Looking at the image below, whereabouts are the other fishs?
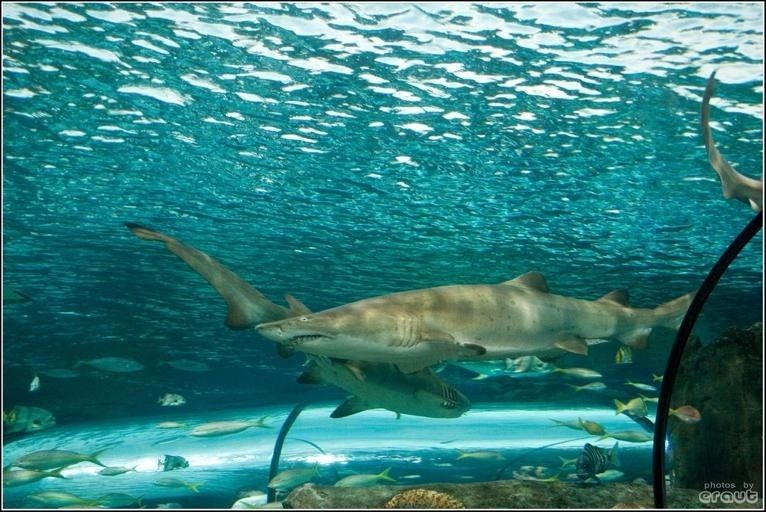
[3,393,208,510]
